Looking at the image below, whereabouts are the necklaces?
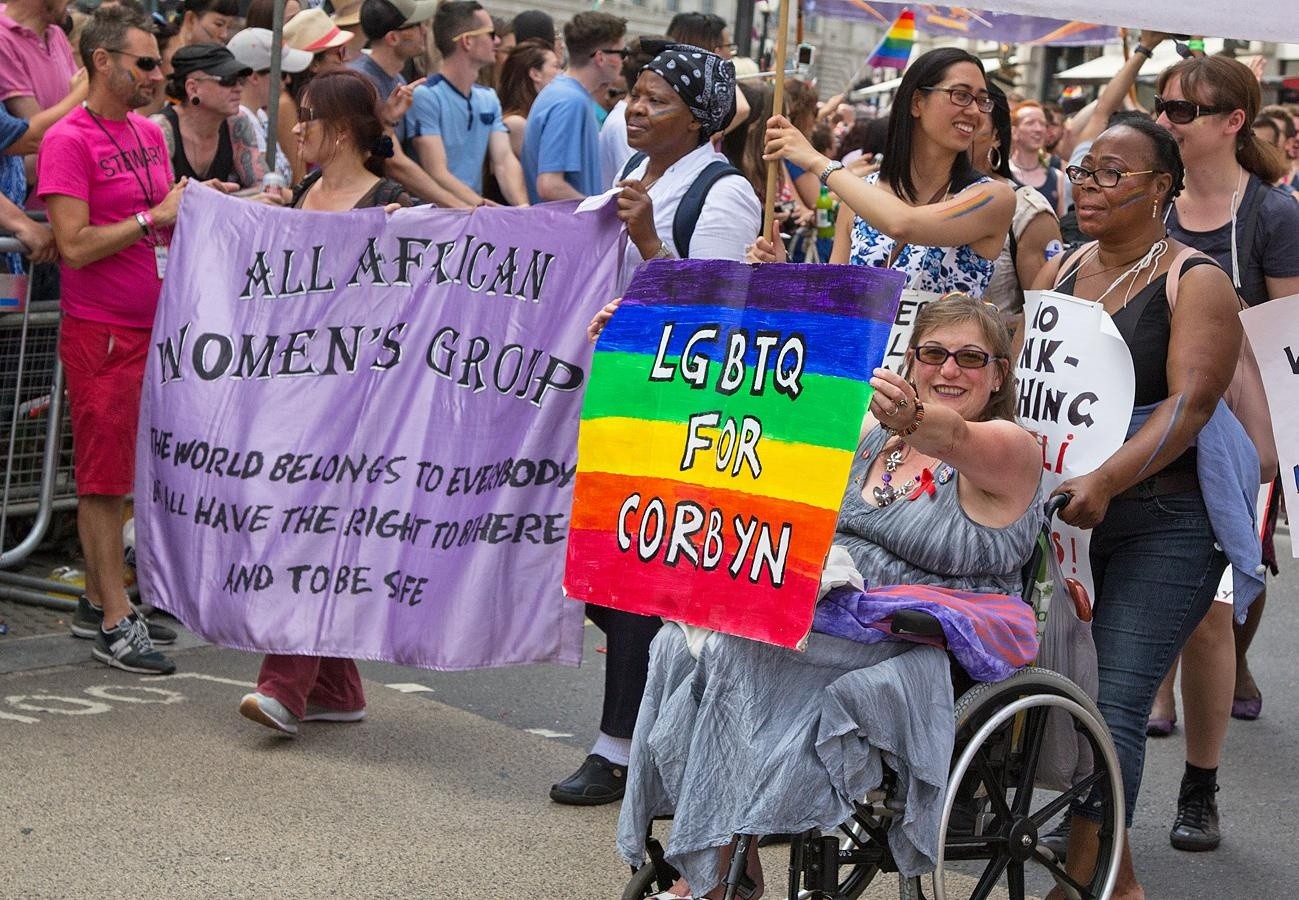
[191,137,210,177]
[1010,157,1040,171]
[872,440,938,506]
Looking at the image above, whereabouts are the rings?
[885,404,899,416]
[894,396,908,408]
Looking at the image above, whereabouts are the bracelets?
[879,396,925,438]
[1188,40,1204,52]
[379,112,400,128]
[1134,45,1153,59]
[142,210,155,229]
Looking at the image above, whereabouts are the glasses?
[920,84,995,113]
[1065,164,1160,188]
[452,27,496,42]
[296,106,325,122]
[911,345,997,368]
[87,47,164,72]
[197,76,244,87]
[589,46,627,60]
[719,42,739,56]
[1154,95,1231,126]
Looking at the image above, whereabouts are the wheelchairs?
[616,487,1127,900]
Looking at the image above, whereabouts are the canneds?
[262,172,286,198]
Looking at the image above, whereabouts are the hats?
[330,0,364,26]
[360,0,437,40]
[282,8,354,53]
[227,28,314,73]
[167,43,253,77]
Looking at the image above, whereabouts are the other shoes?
[1231,689,1262,718]
[1146,713,1177,735]
[551,754,628,806]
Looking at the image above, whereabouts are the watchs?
[135,213,149,236]
[652,241,672,260]
[819,159,845,187]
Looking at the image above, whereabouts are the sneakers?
[1038,807,1072,865]
[297,702,366,722]
[1170,773,1220,851]
[92,613,176,674]
[72,593,177,644]
[239,692,298,736]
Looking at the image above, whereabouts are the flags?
[866,10,916,70]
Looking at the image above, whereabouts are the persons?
[1027,116,1245,900]
[238,65,417,736]
[617,292,1047,899]
[36,5,230,675]
[547,44,764,806]
[0,0,1299,835]
